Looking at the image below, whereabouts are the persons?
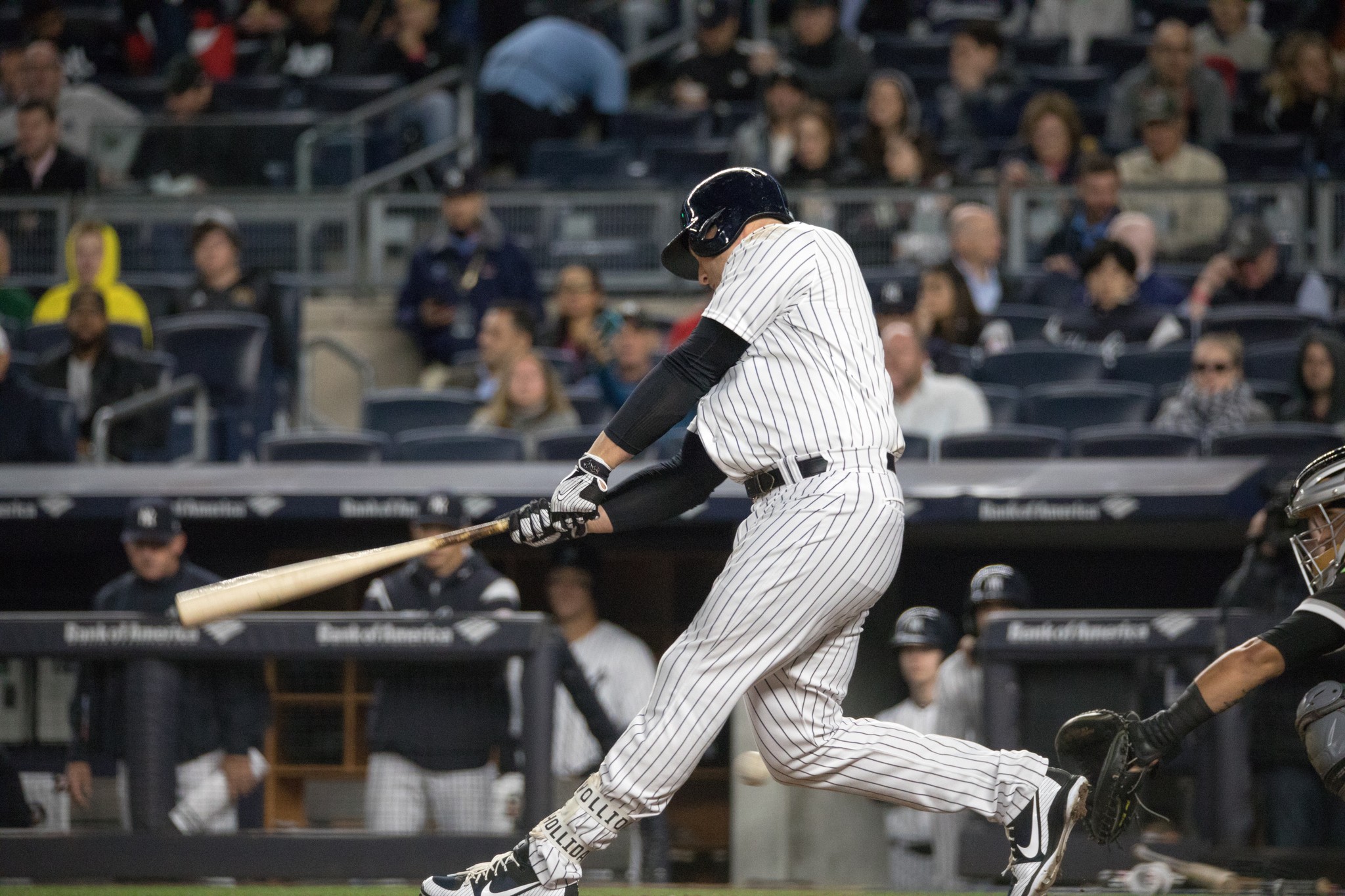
[421,164,1092,896]
[0,0,1345,896]
[62,496,278,833]
[1049,444,1345,896]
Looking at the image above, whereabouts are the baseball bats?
[175,475,610,629]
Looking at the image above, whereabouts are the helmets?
[1284,449,1345,593]
[662,166,794,281]
[969,566,1023,607]
[893,604,952,652]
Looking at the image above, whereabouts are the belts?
[745,452,896,497]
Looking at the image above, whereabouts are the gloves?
[495,499,588,548]
[553,452,615,532]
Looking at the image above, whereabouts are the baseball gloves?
[1055,710,1152,846]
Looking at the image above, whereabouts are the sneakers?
[420,840,579,896]
[1000,765,1093,896]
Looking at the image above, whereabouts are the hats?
[119,496,179,544]
[443,168,480,196]
[410,492,470,530]
